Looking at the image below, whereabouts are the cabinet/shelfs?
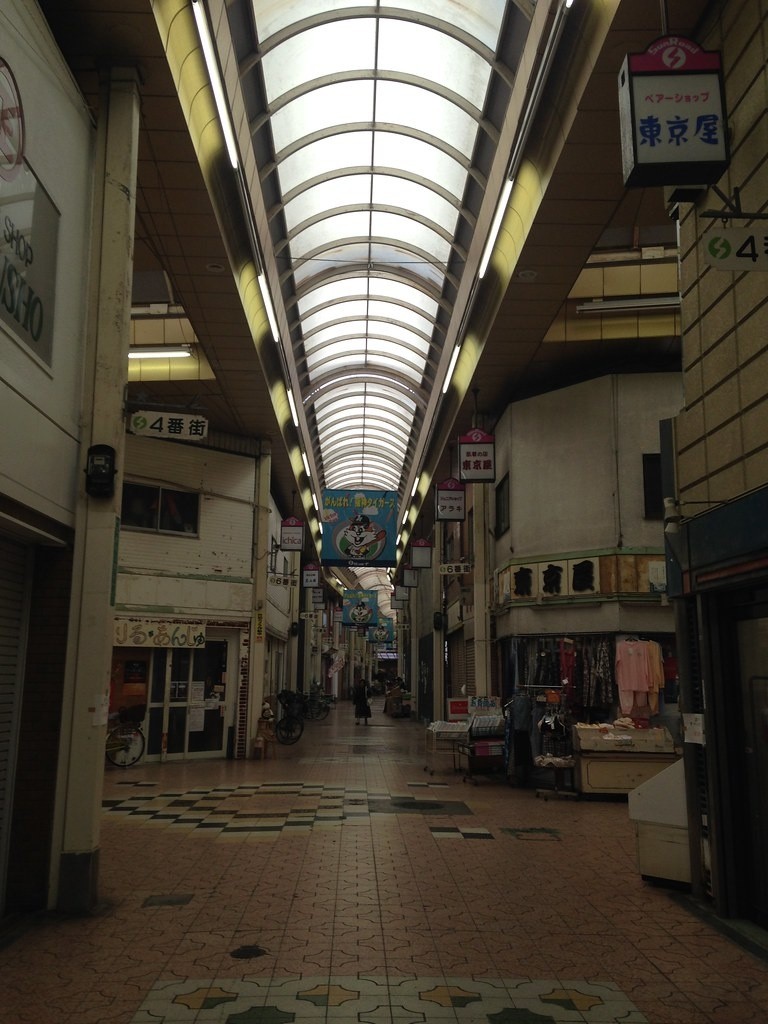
[424,728,504,785]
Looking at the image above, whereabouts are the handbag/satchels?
[367,698,373,706]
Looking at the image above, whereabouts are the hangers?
[582,634,612,643]
[625,633,650,642]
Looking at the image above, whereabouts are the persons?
[386,680,395,690]
[353,679,372,725]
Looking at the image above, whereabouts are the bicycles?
[275,690,330,747]
[106,713,145,768]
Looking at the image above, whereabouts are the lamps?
[84,444,116,502]
[661,494,726,523]
[433,612,443,630]
[292,621,298,636]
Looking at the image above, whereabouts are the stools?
[257,718,276,766]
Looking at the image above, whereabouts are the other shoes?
[356,722,359,725]
[365,722,367,725]
